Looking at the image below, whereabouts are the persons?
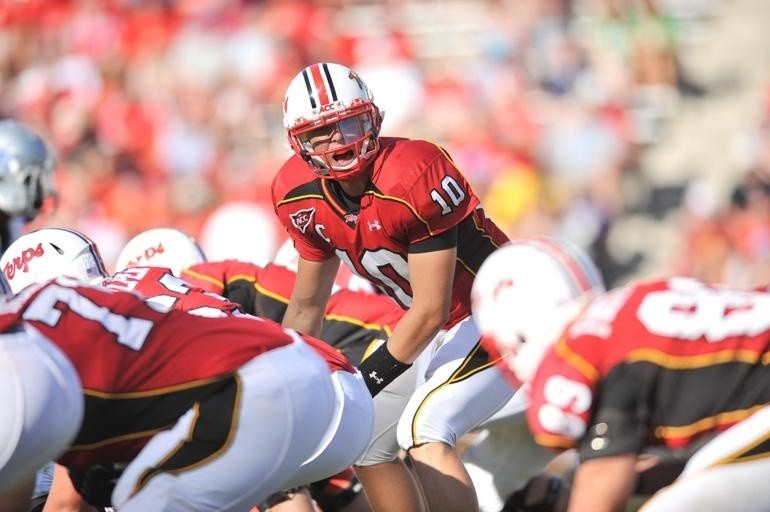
[1,226,377,511]
[267,59,522,512]
[469,234,770,512]
[114,227,430,512]
[0,266,86,512]
[0,2,768,303]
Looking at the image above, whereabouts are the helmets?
[0,119,52,221]
[469,234,608,390]
[0,227,110,302]
[115,227,208,279]
[283,62,381,178]
